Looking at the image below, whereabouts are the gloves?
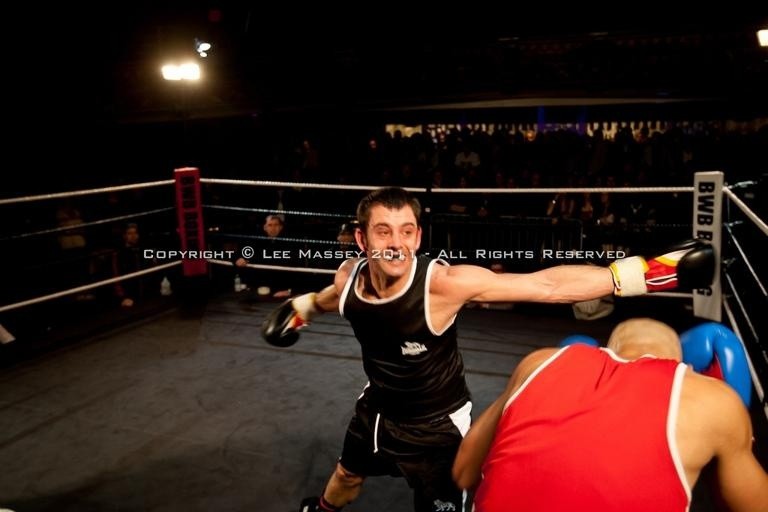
[610,240,716,297]
[681,323,751,405]
[262,292,316,347]
[561,334,598,346]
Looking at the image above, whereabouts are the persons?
[451,318,768,512]
[261,188,715,512]
[57,126,662,344]
